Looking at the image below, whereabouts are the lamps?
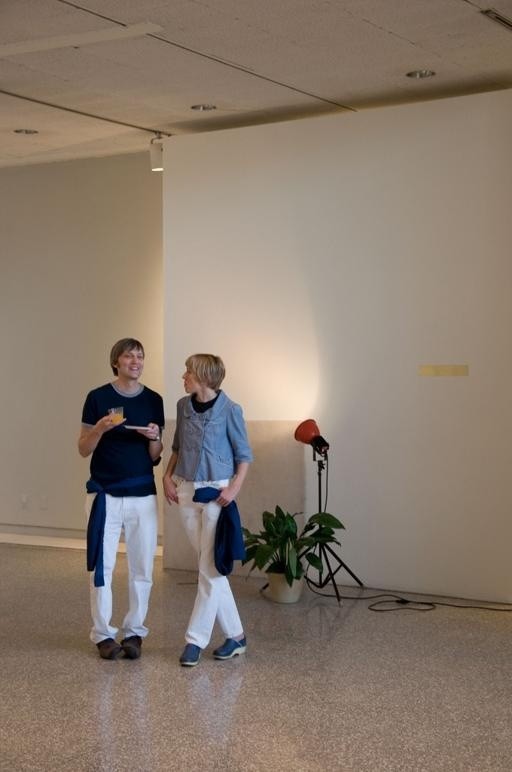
[149,132,163,171]
[259,419,363,602]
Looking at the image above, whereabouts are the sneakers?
[214,635,248,659]
[97,637,122,659]
[179,643,203,666]
[120,635,142,658]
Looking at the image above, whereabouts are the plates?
[125,425,152,431]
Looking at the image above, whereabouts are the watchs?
[150,434,159,441]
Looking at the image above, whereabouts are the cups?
[108,406,124,424]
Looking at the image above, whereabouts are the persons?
[162,354,255,666]
[79,338,166,660]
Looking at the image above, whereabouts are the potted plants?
[240,505,346,601]
